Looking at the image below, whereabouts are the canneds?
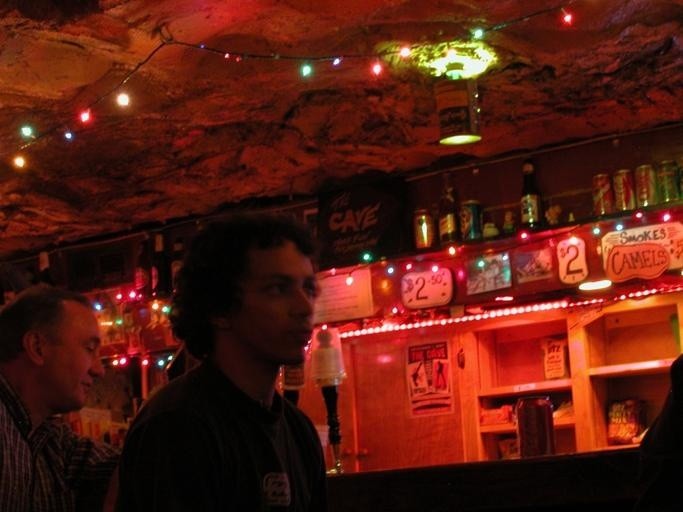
[458,199,482,242]
[515,396,556,458]
[415,209,436,249]
[589,159,682,217]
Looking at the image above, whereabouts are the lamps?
[434,77,483,145]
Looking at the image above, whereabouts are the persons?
[114,209,329,512]
[465,251,511,295]
[0,282,122,510]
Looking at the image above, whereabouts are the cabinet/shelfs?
[334,291,682,476]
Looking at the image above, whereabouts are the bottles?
[31,247,55,289]
[436,169,459,244]
[518,156,542,234]
[132,231,187,303]
